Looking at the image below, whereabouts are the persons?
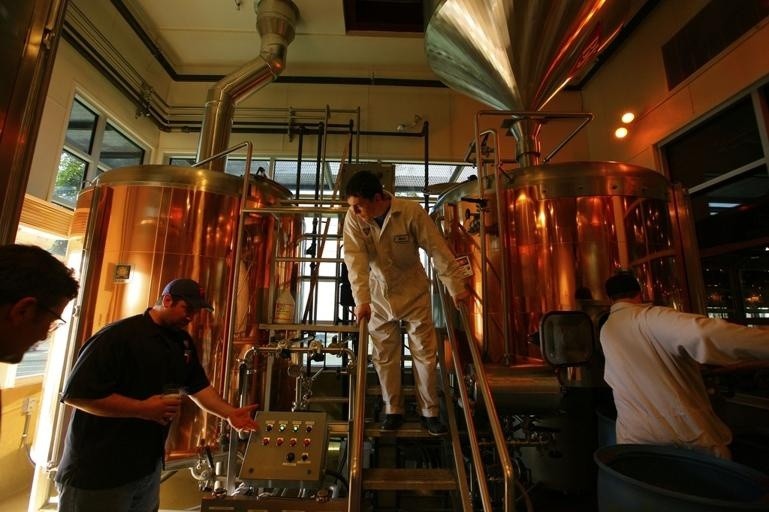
[598,270,769,461]
[574,286,608,445]
[52,278,261,511]
[0,243,80,366]
[342,169,470,437]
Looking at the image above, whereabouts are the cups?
[162,386,182,422]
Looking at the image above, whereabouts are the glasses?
[40,305,67,333]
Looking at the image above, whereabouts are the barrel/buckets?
[592,443,769,512]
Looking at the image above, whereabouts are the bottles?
[273,283,296,325]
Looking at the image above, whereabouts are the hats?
[161,278,214,313]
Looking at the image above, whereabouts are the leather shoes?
[381,414,405,429]
[420,414,450,436]
